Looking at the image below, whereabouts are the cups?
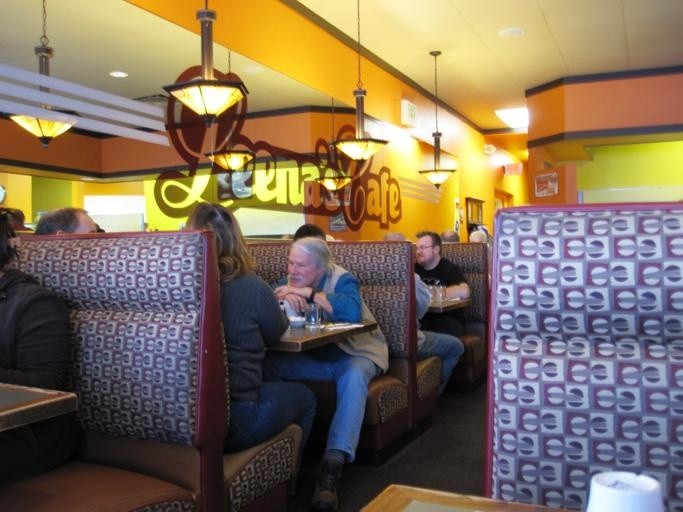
[304,302,322,332]
[280,295,306,331]
[425,283,447,305]
[586,471,664,511]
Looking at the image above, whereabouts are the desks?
[358,484,575,511]
[275,319,379,353]
[1,383,79,433]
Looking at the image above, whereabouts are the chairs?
[485,203,682,511]
[243,240,488,432]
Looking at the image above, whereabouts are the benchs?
[16,230,304,509]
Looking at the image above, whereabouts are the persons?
[385,231,471,393]
[469,226,493,243]
[183,203,318,495]
[0,205,75,483]
[270,237,388,509]
[293,224,326,241]
[35,208,95,235]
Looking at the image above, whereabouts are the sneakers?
[311,458,344,510]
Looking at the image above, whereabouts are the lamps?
[419,51,455,190]
[331,1,389,166]
[162,0,250,128]
[205,50,256,175]
[7,0,81,149]
